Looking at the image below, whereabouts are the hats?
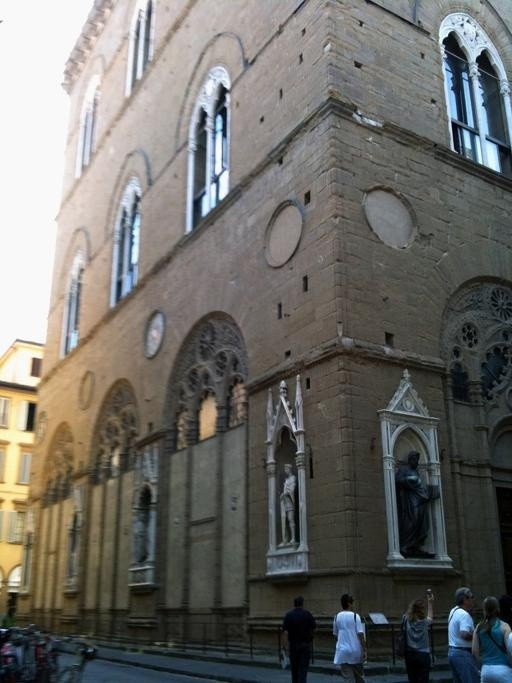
[455,587,474,599]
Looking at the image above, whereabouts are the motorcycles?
[0,624,99,682]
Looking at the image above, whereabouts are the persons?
[445,587,475,683]
[280,595,319,683]
[400,592,435,683]
[394,450,438,556]
[471,596,512,683]
[332,592,369,683]
[277,463,297,547]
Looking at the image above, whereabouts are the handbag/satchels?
[394,634,409,657]
